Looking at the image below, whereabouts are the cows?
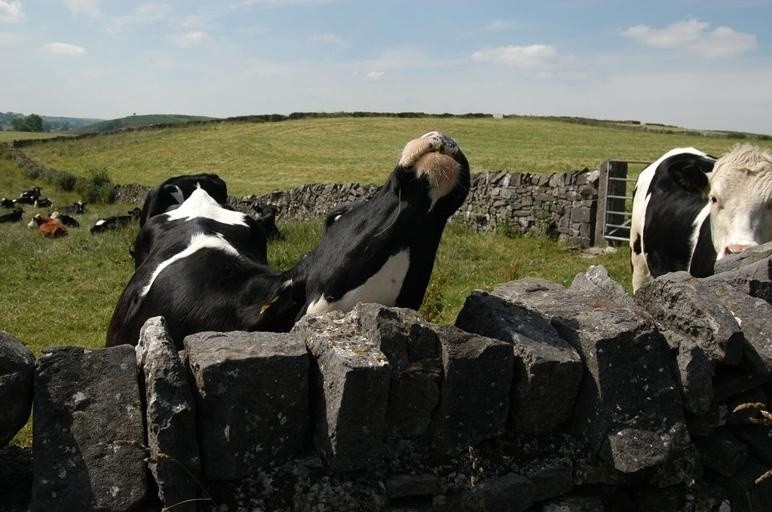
[27,214,69,240]
[104,130,471,348]
[54,200,88,218]
[0,185,56,223]
[629,142,772,295]
[139,172,228,228]
[89,207,142,236]
[50,211,80,231]
